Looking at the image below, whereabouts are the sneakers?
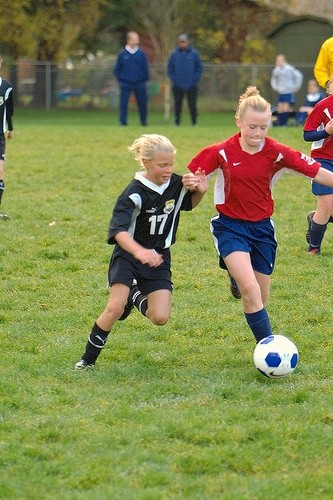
[118,278,138,320]
[228,273,242,299]
[75,360,94,372]
[306,211,316,243]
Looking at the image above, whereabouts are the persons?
[0,57,14,220]
[113,31,149,126]
[270,53,304,127]
[303,93,333,255]
[181,86,333,344]
[296,80,328,126]
[75,134,208,372]
[313,37,333,96]
[168,33,202,127]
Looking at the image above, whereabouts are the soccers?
[254,334,299,378]
